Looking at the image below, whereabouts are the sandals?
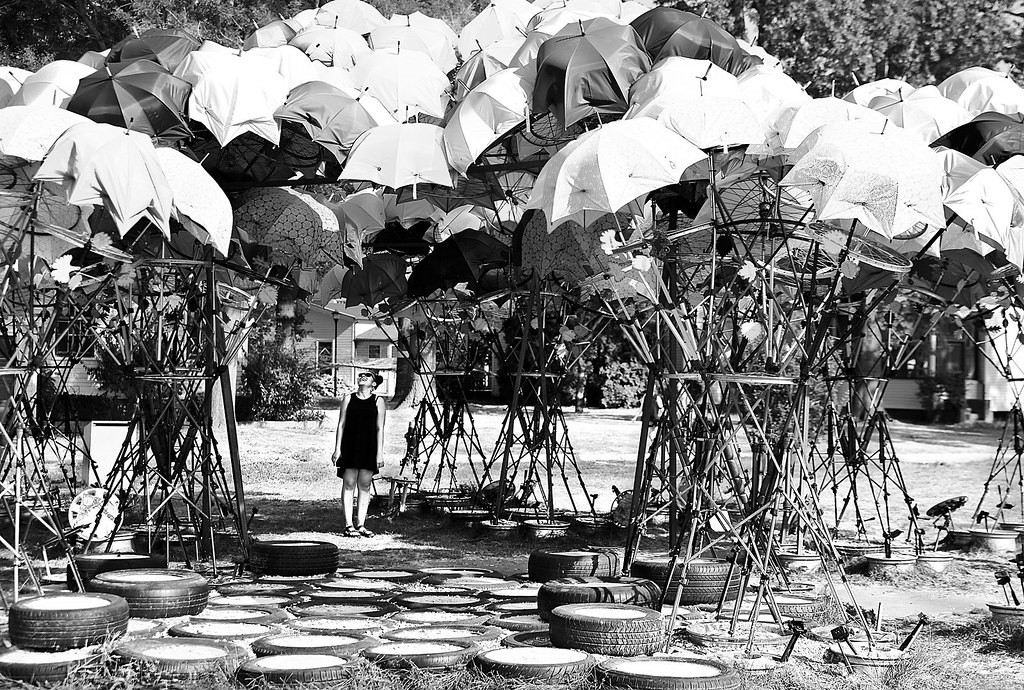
[343,524,361,538]
[355,525,375,538]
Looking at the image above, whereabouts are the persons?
[332,370,383,536]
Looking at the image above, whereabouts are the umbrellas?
[1,0,1023,319]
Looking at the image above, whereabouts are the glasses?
[358,372,376,382]
